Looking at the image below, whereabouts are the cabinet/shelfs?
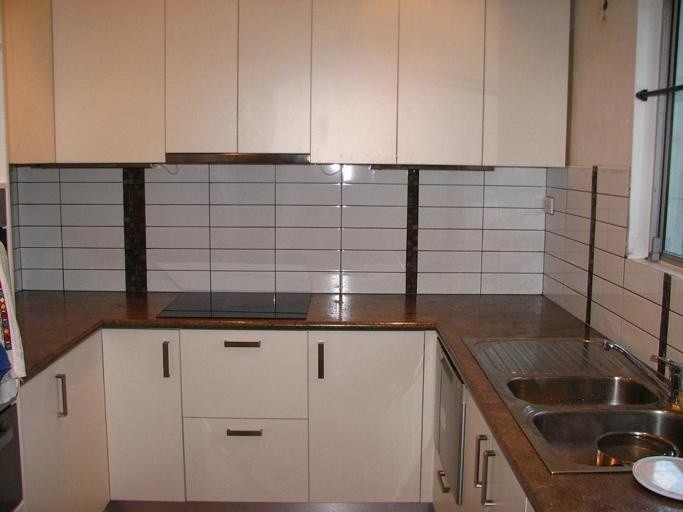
[397,1,570,169]
[180,326,309,503]
[101,327,186,502]
[165,1,312,153]
[15,327,109,512]
[420,329,533,512]
[309,2,399,165]
[308,328,425,503]
[2,2,166,164]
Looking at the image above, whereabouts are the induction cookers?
[154,292,312,320]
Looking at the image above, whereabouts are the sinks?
[532,409,683,466]
[508,374,662,405]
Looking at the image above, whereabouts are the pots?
[593,430,680,467]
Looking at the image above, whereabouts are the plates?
[632,454,683,502]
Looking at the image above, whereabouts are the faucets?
[601,340,683,403]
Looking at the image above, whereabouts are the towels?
[0,239,26,407]
